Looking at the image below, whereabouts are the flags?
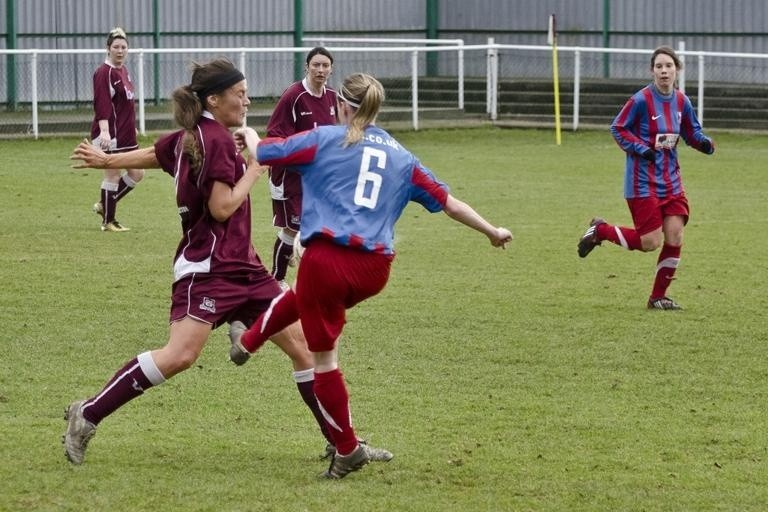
[547,15,555,47]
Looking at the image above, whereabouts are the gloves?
[642,149,655,164]
[698,139,711,154]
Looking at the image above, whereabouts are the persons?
[578,46,713,307]
[63,59,396,467]
[255,45,346,291]
[91,26,144,234]
[228,74,512,479]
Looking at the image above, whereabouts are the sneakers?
[319,445,370,479]
[93,203,105,217]
[288,234,302,267]
[578,217,608,258]
[61,400,97,466]
[229,320,251,366]
[101,221,129,231]
[277,280,290,292]
[647,297,681,310]
[325,435,394,461]
